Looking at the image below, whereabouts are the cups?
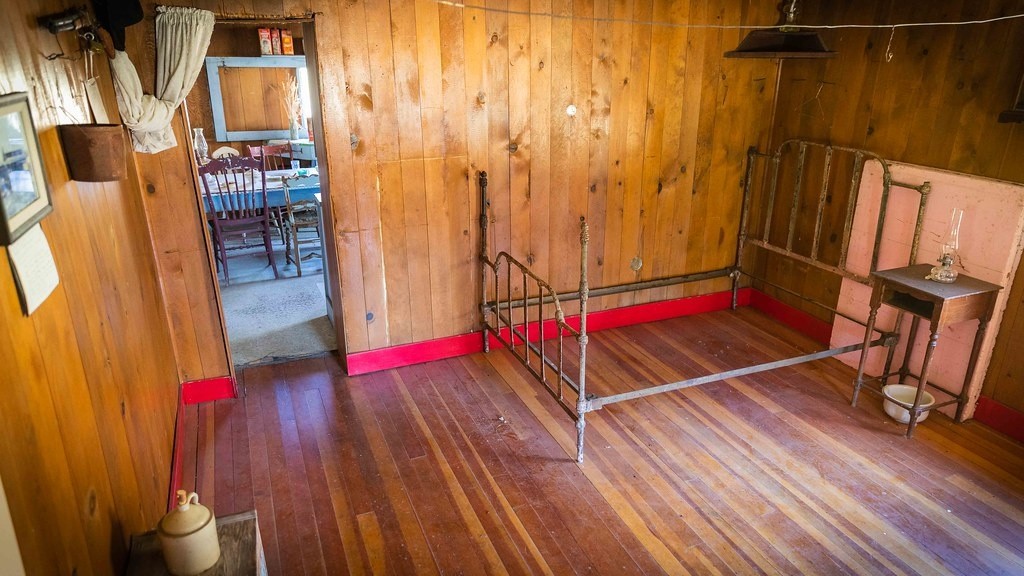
[291,160,300,171]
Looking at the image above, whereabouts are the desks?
[268,137,318,171]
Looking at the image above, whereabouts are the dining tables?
[196,167,322,216]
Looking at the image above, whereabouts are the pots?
[155,488,221,574]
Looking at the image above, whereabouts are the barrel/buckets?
[58,105,130,182]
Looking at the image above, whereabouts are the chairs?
[198,149,282,288]
[201,142,282,247]
[279,174,325,277]
[248,142,321,243]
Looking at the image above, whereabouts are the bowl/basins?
[880,382,936,423]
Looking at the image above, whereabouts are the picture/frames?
[0,89,55,246]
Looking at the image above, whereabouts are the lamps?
[721,1,842,62]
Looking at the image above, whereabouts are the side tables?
[850,262,1007,440]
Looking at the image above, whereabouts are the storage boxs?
[54,80,129,183]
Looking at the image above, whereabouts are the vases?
[192,127,209,161]
[288,117,299,140]
